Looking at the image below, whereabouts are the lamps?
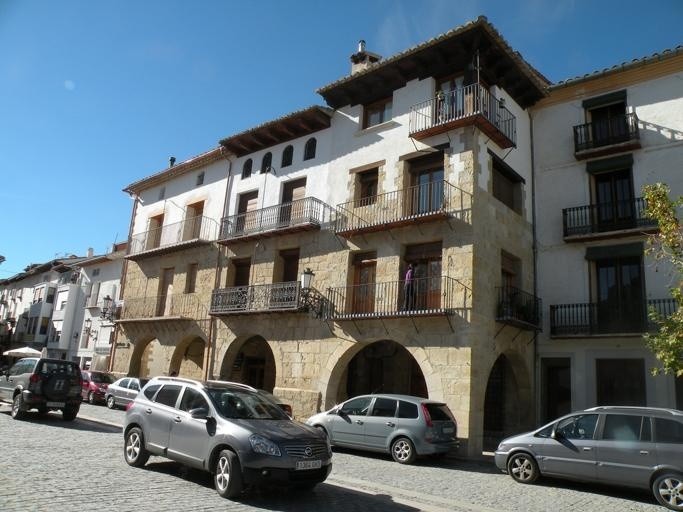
[83,318,97,342]
[99,295,119,329]
[300,267,328,320]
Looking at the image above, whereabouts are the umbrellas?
[2,346,40,359]
[41,347,48,372]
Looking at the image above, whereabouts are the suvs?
[0,357,83,422]
[123,375,332,497]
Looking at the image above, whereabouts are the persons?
[400,262,417,310]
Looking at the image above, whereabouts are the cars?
[492,406,683,511]
[81,370,118,405]
[304,393,460,462]
[105,376,149,409]
